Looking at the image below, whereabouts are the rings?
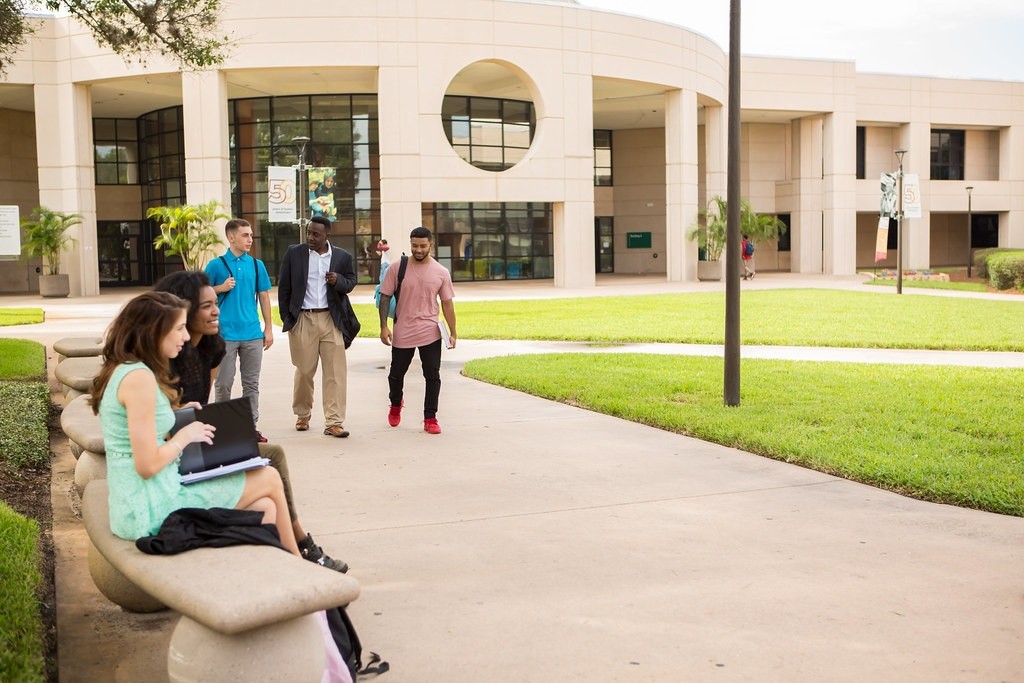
[327,279,328,281]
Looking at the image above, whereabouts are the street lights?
[291,135,311,245]
[894,147,907,293]
[965,186,974,278]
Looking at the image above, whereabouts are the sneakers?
[423,418,441,433]
[254,431,267,443]
[298,533,348,574]
[389,401,405,426]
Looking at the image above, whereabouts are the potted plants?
[739,206,787,278]
[145,200,232,277]
[686,195,758,282]
[11,206,84,298]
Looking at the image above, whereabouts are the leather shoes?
[324,426,348,437]
[296,414,311,430]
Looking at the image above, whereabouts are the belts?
[301,307,329,313]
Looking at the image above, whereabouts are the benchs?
[52,335,359,683]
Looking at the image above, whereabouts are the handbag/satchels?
[373,253,409,317]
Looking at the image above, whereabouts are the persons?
[376,239,390,284]
[84,291,304,560]
[378,227,456,434]
[203,218,274,443]
[308,169,338,222]
[277,216,361,438]
[152,270,349,575]
[740,234,757,282]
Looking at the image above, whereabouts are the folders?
[169,396,266,485]
